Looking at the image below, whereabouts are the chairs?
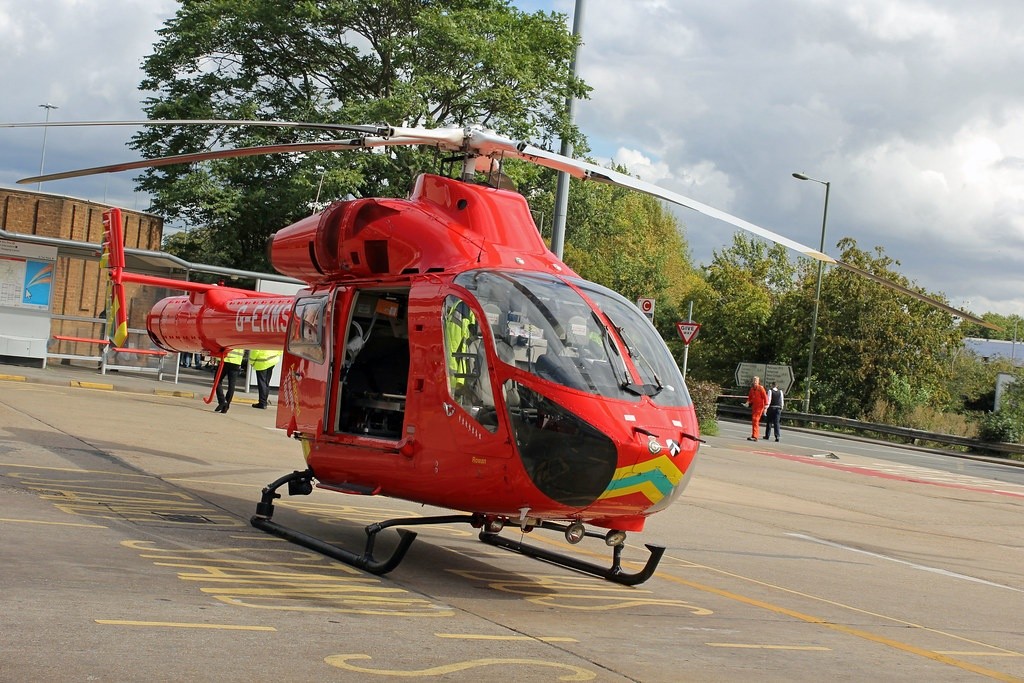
[357,336,410,418]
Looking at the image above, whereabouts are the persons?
[214,349,245,413]
[763,382,784,442]
[184,353,204,370]
[745,375,768,441]
[248,350,283,409]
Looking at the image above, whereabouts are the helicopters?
[0,121,1009,591]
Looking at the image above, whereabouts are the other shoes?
[220,403,229,413]
[763,436,769,440]
[747,437,758,442]
[252,402,267,409]
[215,399,227,411]
[775,439,779,442]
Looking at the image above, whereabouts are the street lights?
[787,165,830,412]
[38,103,59,193]
